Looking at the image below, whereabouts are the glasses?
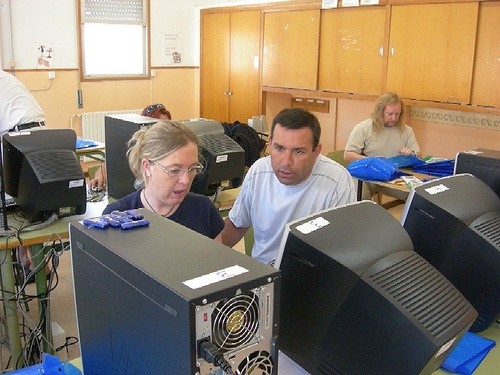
[145,105,162,115]
[151,160,204,176]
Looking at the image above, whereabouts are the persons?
[101,120,226,245]
[213,108,358,271]
[89,103,174,191]
[343,93,422,201]
[0,67,52,285]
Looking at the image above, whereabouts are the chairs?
[326,149,349,167]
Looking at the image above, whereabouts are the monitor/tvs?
[3,129,86,223]
[400,172,500,332]
[181,118,246,196]
[274,200,477,375]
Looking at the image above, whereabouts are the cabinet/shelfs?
[260,0,500,115]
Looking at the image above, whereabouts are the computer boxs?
[105,113,161,199]
[453,147,500,197]
[68,207,282,375]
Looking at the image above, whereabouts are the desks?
[0,184,243,369]
[358,168,438,201]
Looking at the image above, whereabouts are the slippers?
[11,255,30,269]
[14,268,51,284]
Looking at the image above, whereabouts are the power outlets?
[49,72,55,79]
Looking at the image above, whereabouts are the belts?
[9,123,46,132]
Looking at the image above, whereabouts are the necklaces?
[143,188,177,217]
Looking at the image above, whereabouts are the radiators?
[71,110,143,142]
